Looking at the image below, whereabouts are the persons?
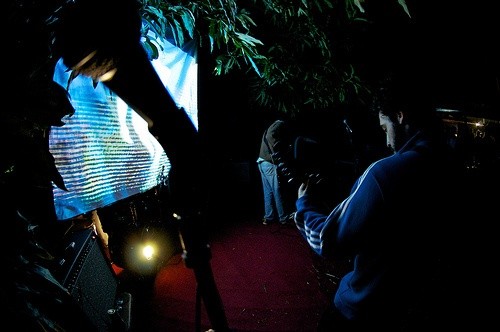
[293,72,499,332]
[256,108,296,225]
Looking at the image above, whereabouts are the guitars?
[270,138,359,301]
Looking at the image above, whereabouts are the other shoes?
[261,218,271,225]
[280,214,290,224]
